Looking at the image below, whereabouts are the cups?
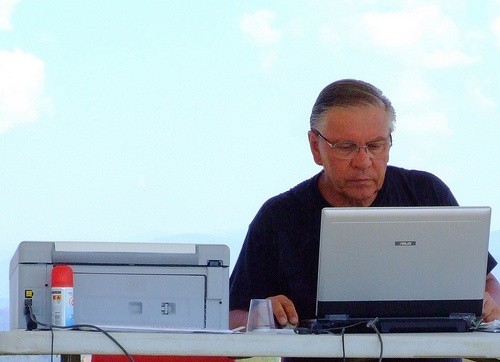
[247,299,275,332]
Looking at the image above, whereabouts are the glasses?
[311,127,392,159]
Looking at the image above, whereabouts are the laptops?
[300,206,492,322]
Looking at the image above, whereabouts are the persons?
[227,79,499,332]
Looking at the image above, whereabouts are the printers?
[9,241,231,332]
